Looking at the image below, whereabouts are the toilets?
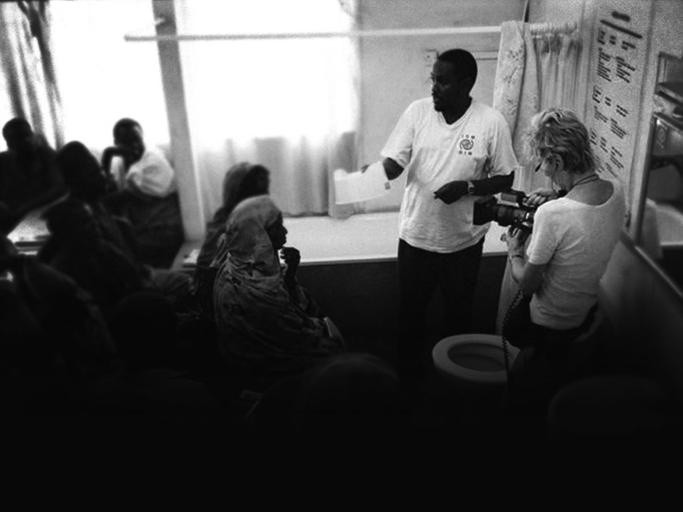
[432,333,520,389]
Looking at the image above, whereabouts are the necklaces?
[575,178,599,186]
[568,174,600,192]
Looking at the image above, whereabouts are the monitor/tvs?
[634,113,683,298]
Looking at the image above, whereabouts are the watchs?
[467,178,474,197]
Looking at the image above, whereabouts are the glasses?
[534,160,543,175]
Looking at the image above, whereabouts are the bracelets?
[507,254,521,264]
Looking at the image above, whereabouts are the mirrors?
[630,51,683,300]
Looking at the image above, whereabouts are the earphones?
[555,161,561,169]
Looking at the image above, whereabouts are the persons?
[499,106,627,449]
[359,46,515,387]
[0,117,407,510]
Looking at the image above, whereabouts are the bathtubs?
[277,211,512,266]
[654,204,683,246]
